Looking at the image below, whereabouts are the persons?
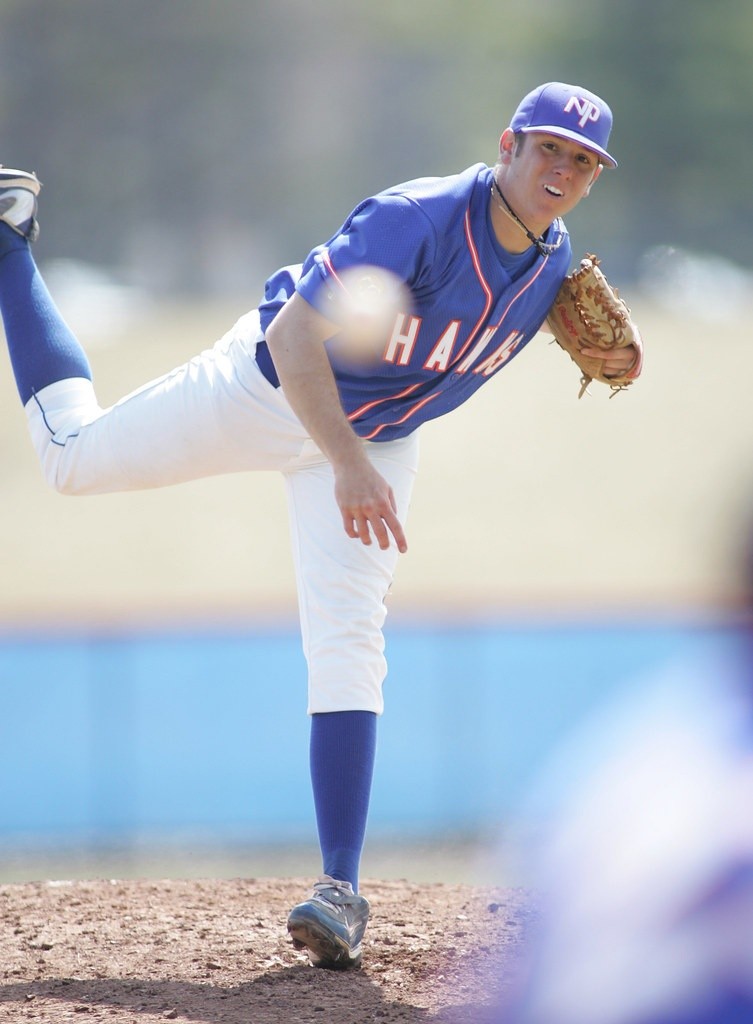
[0,81,642,969]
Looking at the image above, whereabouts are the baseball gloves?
[549,254,641,399]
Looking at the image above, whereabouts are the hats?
[509,81,618,170]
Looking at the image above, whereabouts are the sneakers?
[0,169,42,242]
[287,874,369,971]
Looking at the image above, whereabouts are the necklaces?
[488,178,565,256]
